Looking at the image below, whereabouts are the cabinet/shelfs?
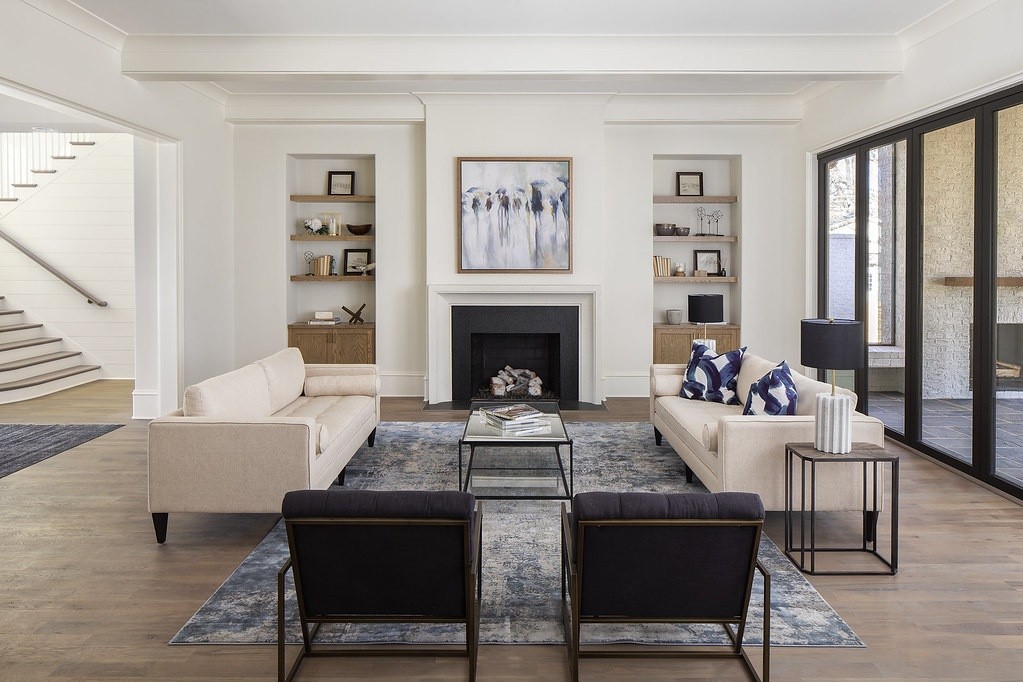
[653,195,738,283]
[290,194,376,281]
[288,320,374,365]
[653,321,740,364]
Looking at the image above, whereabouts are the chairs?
[561,491,772,682]
[278,488,482,682]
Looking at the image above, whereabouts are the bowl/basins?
[346,224,372,236]
[655,223,677,236]
[675,227,690,237]
[667,309,683,325]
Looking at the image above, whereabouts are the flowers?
[302,218,330,236]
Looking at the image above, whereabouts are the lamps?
[687,294,723,358]
[800,318,863,455]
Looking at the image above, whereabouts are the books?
[308,312,339,325]
[485,403,543,428]
[653,256,670,276]
[313,255,334,275]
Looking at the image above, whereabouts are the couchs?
[146,346,379,545]
[649,353,884,544]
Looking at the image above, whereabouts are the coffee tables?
[459,398,574,508]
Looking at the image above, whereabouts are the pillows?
[679,340,747,406]
[743,358,798,415]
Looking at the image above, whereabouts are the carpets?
[0,422,127,479]
[166,422,865,646]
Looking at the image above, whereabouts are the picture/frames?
[677,171,703,197]
[694,250,721,276]
[328,171,355,196]
[345,248,370,275]
[457,157,572,275]
